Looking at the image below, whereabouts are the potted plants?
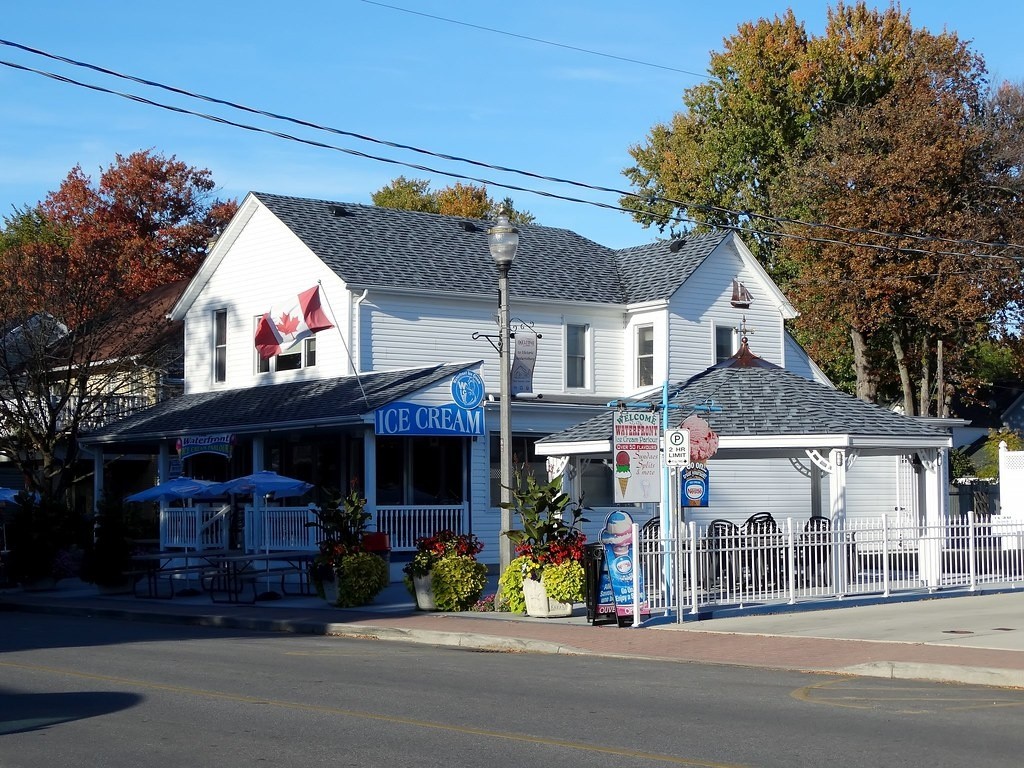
[54,491,143,595]
[305,477,389,608]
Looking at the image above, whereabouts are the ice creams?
[176,440,181,459]
[600,511,634,558]
[680,417,719,506]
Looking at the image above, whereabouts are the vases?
[524,575,573,618]
[412,571,437,610]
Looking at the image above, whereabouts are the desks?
[211,550,320,605]
[133,548,247,598]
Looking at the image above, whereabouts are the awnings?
[0,480,48,552]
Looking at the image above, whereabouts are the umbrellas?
[125,468,310,592]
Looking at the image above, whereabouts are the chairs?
[635,512,831,592]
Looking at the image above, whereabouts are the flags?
[241,285,335,360]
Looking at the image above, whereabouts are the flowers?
[497,453,589,615]
[402,528,488,613]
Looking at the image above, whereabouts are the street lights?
[486,202,521,612]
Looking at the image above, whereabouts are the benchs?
[137,563,308,604]
